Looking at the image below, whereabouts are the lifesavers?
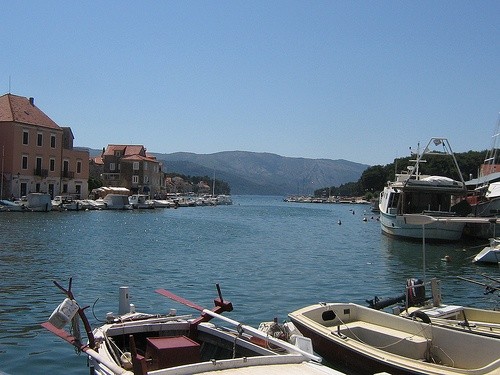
[409,310,432,325]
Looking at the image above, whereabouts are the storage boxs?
[144,335,200,370]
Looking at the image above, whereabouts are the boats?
[283,138,500,263]
[0,193,233,212]
[365,278,500,338]
[41,274,347,375]
[287,301,500,375]
